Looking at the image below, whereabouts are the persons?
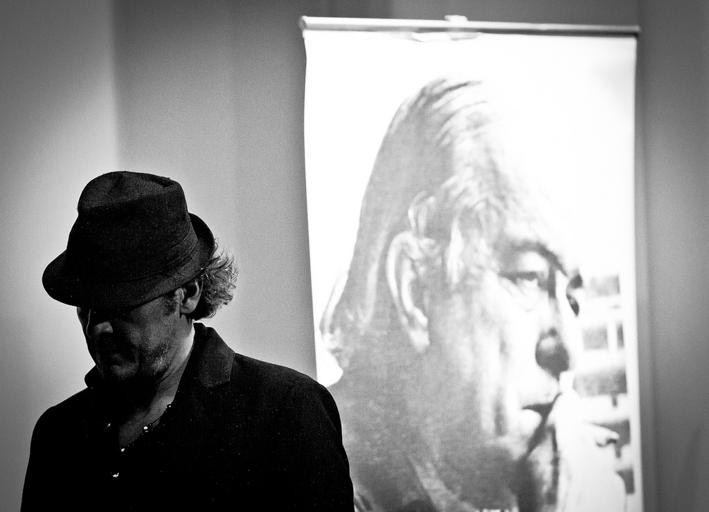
[21,172,353,511]
[318,69,629,511]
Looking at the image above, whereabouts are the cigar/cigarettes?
[589,424,620,448]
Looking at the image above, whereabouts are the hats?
[42,170,214,308]
